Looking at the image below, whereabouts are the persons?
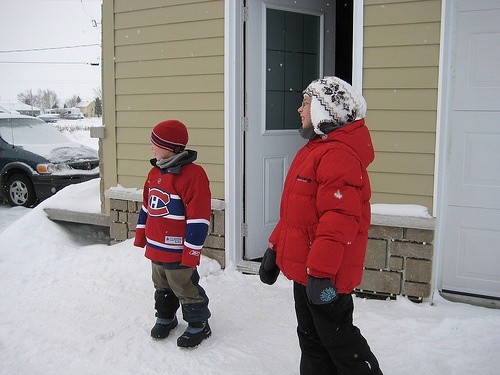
[258,76,384,375]
[133,119,211,349]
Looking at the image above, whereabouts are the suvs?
[0,117,99,207]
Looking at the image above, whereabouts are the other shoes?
[151,313,178,339]
[176,320,212,347]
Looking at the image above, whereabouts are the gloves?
[260,245,282,285]
[305,275,338,305]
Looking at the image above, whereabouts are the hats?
[151,119,189,154]
[303,77,366,136]
